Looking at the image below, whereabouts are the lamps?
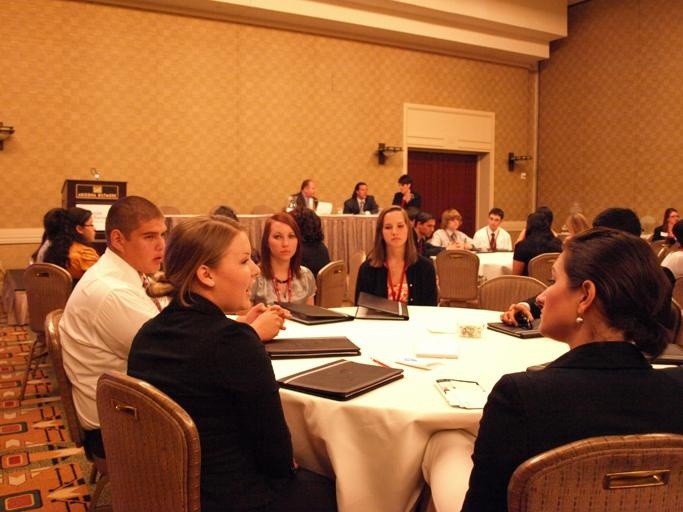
[0,121,16,150]
[508,152,532,172]
[378,141,402,165]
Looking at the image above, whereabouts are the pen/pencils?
[369,357,392,368]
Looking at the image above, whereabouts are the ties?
[490,234,496,249]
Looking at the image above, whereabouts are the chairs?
[1,201,683,512]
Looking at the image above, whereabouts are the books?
[280,304,354,325]
[255,335,360,359]
[354,293,409,321]
[488,318,546,339]
[270,358,407,401]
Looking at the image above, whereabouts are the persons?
[391,175,421,209]
[408,207,590,277]
[58,196,291,460]
[217,206,330,305]
[502,207,641,328]
[291,179,318,210]
[353,207,438,306]
[342,182,378,216]
[653,207,683,278]
[127,213,332,511]
[420,226,681,512]
[32,206,100,280]
